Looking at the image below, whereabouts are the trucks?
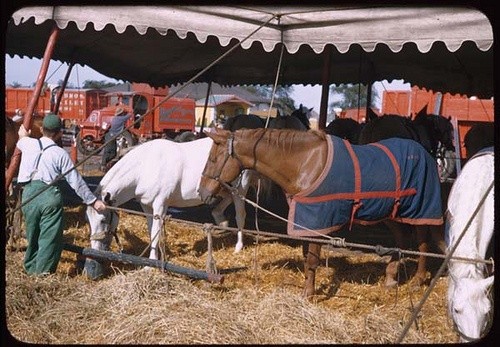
[336,86,494,179]
[75,81,198,157]
[5,86,110,147]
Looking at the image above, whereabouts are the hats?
[43,114,61,129]
[114,108,124,115]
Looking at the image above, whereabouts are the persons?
[99,108,133,171]
[16,114,106,275]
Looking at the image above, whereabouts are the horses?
[197,124,443,305]
[117,105,494,170]
[85,137,269,269]
[444,146,494,344]
[5,112,63,168]
